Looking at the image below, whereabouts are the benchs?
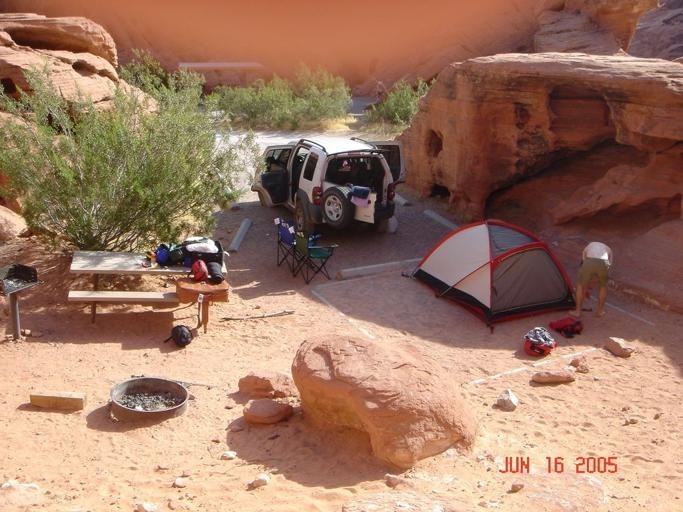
[67,287,210,303]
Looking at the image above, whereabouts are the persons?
[568,241,614,319]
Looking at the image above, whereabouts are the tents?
[410,218,577,334]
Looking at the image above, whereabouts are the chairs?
[276,218,307,271]
[292,228,339,284]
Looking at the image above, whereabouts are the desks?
[70,249,228,334]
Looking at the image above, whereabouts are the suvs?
[251,136,406,236]
